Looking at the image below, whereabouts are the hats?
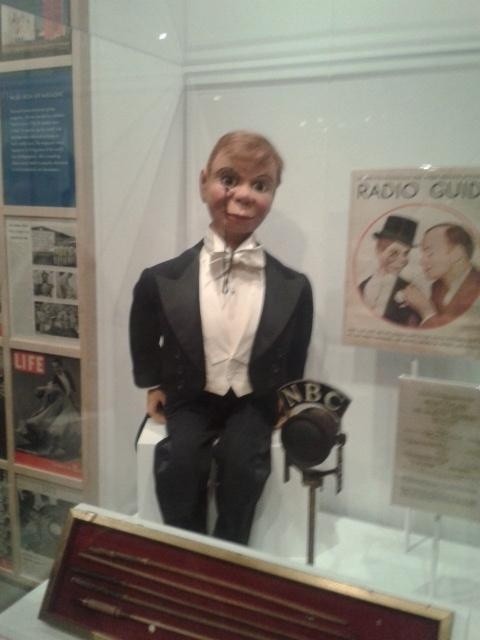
[374,216,416,247]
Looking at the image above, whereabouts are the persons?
[358,210,424,332]
[124,128,318,549]
[25,357,79,460]
[404,222,479,332]
